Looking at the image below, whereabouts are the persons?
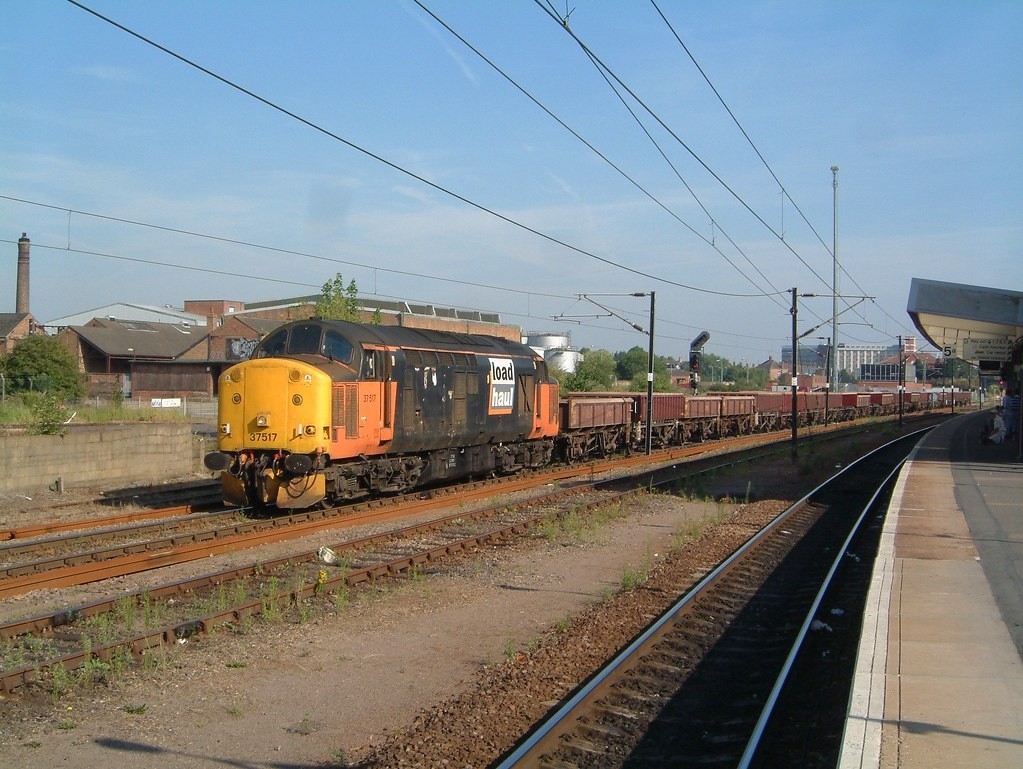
[980,410,1006,447]
[987,388,1021,441]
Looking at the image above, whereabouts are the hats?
[991,409,998,412]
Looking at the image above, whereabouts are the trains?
[203,313,973,514]
